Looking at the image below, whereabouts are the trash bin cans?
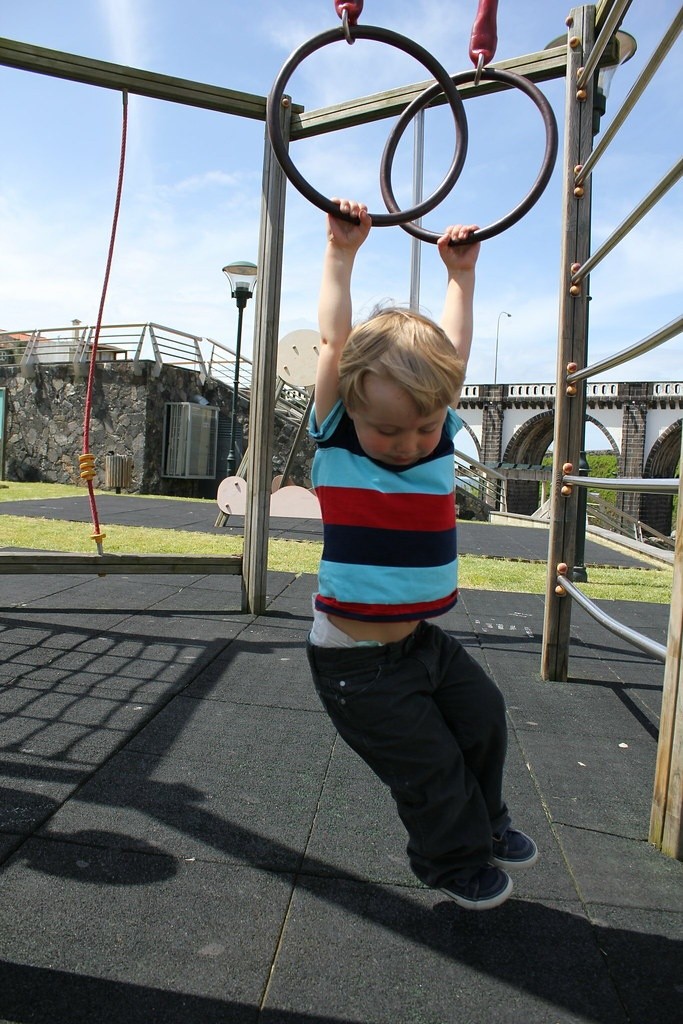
[105,454,131,489]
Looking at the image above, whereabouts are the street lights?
[221,260,257,476]
[494,311,512,386]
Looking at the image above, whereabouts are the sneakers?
[486,818,538,870]
[436,862,513,911]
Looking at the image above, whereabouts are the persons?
[305,197,538,908]
